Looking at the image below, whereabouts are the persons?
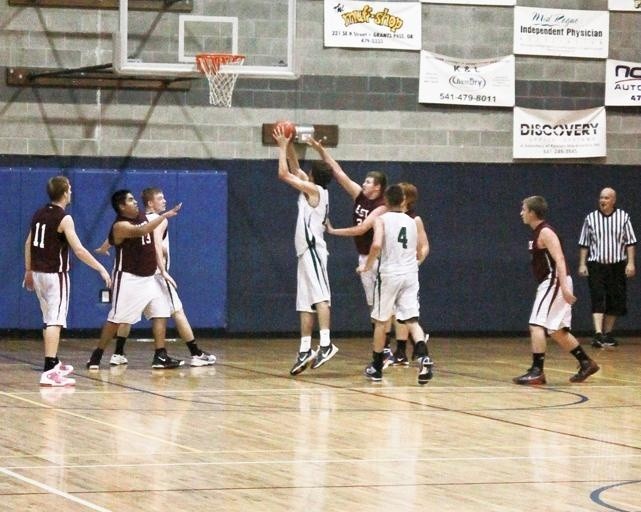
[578,187,637,351]
[388,182,431,366]
[96,187,217,367]
[22,175,112,385]
[512,195,600,384]
[272,124,339,376]
[306,136,397,376]
[86,189,186,370]
[355,184,434,385]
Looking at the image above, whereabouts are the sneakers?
[361,362,382,384]
[591,332,603,347]
[417,356,433,385]
[289,348,318,376]
[602,332,617,346]
[569,359,599,383]
[38,369,75,388]
[512,367,545,385]
[381,346,394,370]
[108,353,128,365]
[310,342,339,369]
[52,359,73,377]
[189,349,216,367]
[86,349,102,370]
[151,351,185,369]
[411,332,430,360]
[389,353,410,366]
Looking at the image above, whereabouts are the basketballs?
[271,121,295,143]
